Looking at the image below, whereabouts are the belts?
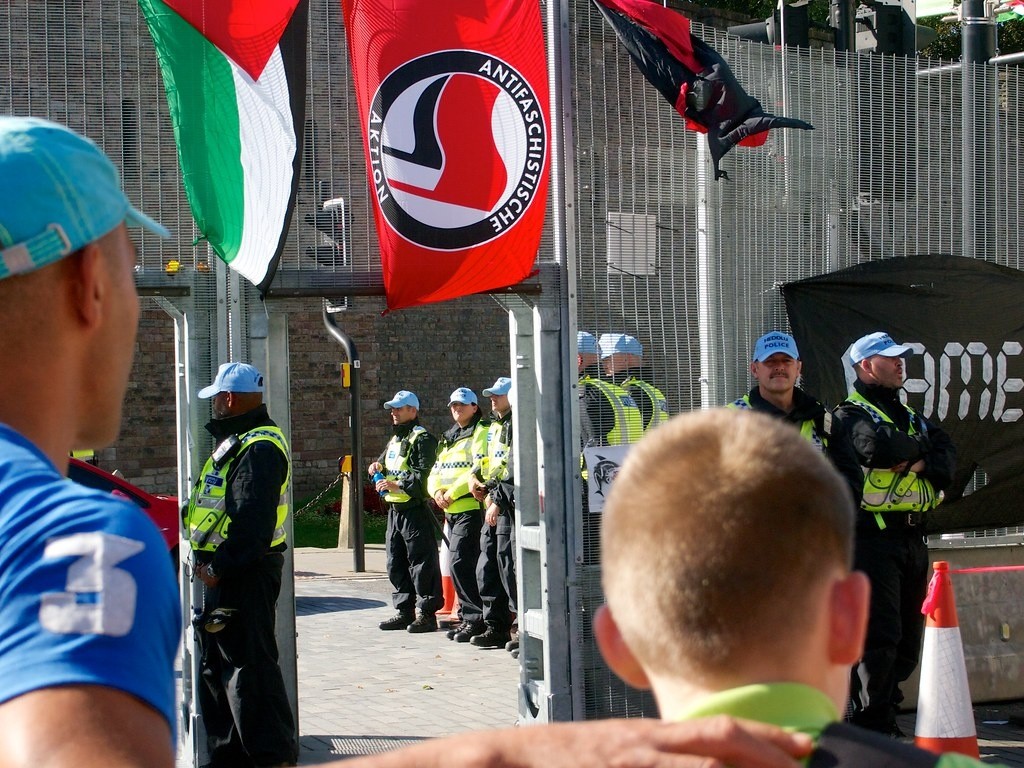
[881,510,927,526]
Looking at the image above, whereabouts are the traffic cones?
[437,517,455,614]
[912,561,980,761]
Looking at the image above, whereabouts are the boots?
[454,619,485,641]
[470,625,513,648]
[381,607,416,630]
[407,610,438,633]
[448,619,468,640]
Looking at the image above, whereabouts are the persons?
[426,388,487,642]
[576,331,670,720]
[183,363,297,768]
[593,409,1010,768]
[833,332,950,737]
[0,116,810,768]
[468,377,520,661]
[727,332,865,513]
[368,390,444,634]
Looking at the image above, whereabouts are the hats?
[753,331,799,363]
[447,387,477,407]
[850,331,913,368]
[383,390,419,411]
[482,376,511,397]
[577,331,603,354]
[198,361,264,397]
[0,114,170,282]
[625,334,643,356]
[598,333,629,359]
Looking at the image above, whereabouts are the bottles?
[374,470,389,497]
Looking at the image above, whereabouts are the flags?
[136,0,310,296]
[593,0,815,182]
[341,0,551,317]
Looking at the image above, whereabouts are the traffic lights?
[854,5,939,201]
[727,0,811,206]
[305,200,357,313]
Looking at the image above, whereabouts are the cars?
[68,456,178,568]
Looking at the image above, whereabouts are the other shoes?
[504,634,519,652]
[867,712,906,736]
